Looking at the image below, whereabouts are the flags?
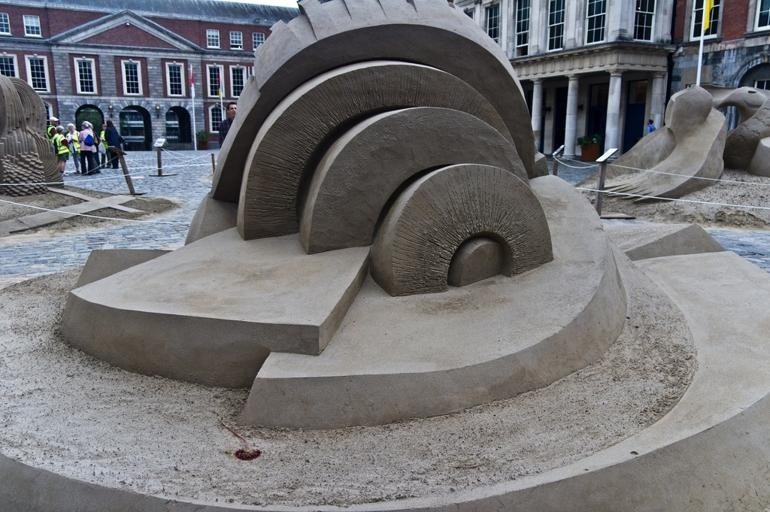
[704,1,714,32]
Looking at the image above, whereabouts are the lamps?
[154,103,160,119]
[108,104,113,118]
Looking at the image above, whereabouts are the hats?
[51,117,59,122]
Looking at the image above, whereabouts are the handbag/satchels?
[98,142,106,154]
[84,134,95,146]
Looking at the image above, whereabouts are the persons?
[218,103,237,149]
[647,120,656,133]
[47,120,124,175]
